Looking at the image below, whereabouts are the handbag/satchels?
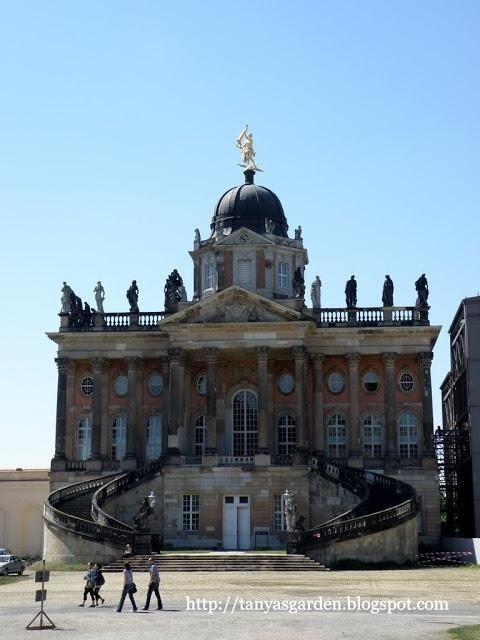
[96,569,105,585]
[124,582,137,594]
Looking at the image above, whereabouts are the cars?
[0,555,26,575]
[0,548,12,555]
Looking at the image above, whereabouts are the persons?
[344,274,357,307]
[193,228,201,252]
[94,281,105,312]
[94,564,104,606]
[311,275,322,307]
[113,562,137,612]
[293,266,306,298]
[77,562,94,607]
[264,217,271,233]
[164,268,187,313]
[215,220,221,232]
[236,132,263,171]
[127,280,139,312]
[268,220,275,234]
[60,281,73,312]
[416,274,429,305]
[381,275,395,307]
[141,557,163,610]
[214,221,225,242]
[149,491,156,507]
[84,301,89,310]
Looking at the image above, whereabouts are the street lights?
[283,488,292,531]
[147,490,157,510]
